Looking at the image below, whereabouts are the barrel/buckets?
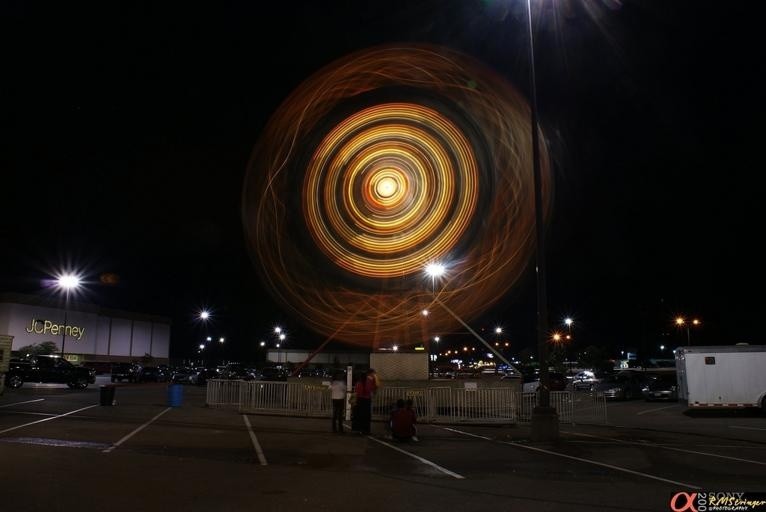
[166,383,184,408]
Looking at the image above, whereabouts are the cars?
[110,361,348,385]
[3,354,97,391]
[523,370,677,402]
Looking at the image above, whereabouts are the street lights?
[56,269,82,358]
[564,316,574,334]
[676,315,700,344]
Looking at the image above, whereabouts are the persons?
[349,368,379,435]
[328,376,346,433]
[389,399,419,442]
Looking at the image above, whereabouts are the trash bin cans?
[168,384,182,407]
[99,384,115,406]
